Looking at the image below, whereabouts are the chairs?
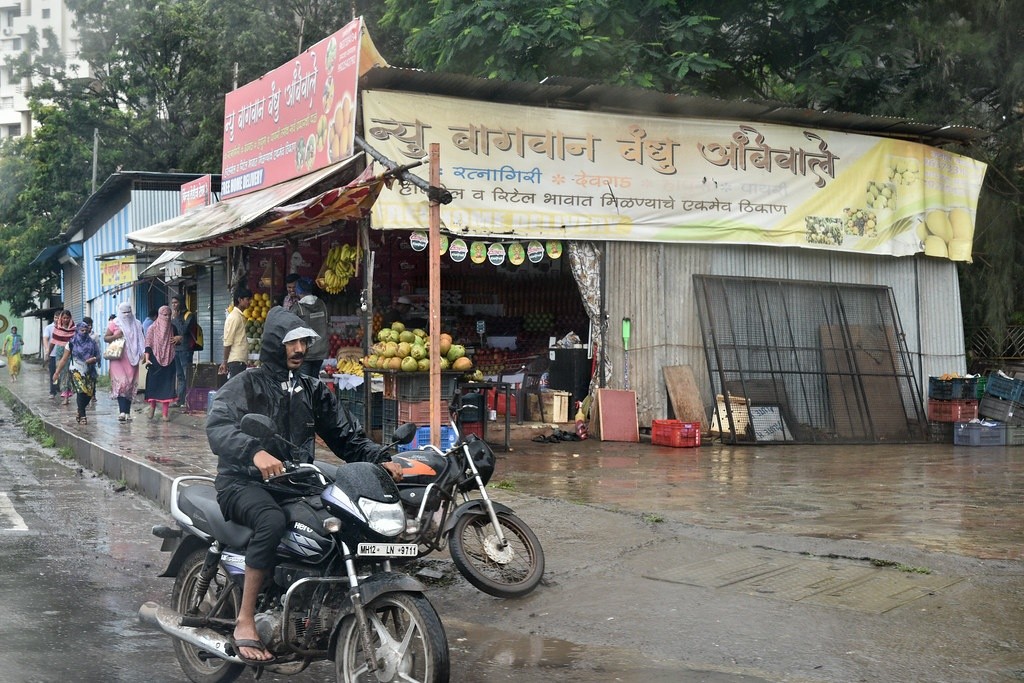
[494,357,551,425]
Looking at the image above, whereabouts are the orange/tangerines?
[358,314,383,336]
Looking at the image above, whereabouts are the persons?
[218,288,252,378]
[144,306,183,422]
[108,314,116,321]
[206,305,406,666]
[284,275,300,312]
[53,322,101,424]
[172,294,195,408]
[2,325,25,384]
[46,309,77,405]
[104,302,146,422]
[290,278,330,381]
[83,317,102,402]
[142,310,158,336]
[44,311,60,399]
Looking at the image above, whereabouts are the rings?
[173,341,174,343]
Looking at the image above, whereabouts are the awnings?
[123,153,429,260]
[29,241,83,272]
[138,249,224,278]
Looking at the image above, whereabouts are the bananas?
[337,358,364,378]
[322,244,364,295]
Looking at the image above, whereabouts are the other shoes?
[149,407,155,419]
[62,397,68,405]
[119,412,132,422]
[76,411,87,425]
[48,394,55,399]
[163,416,169,422]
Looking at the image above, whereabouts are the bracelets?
[94,357,96,359]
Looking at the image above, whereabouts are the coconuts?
[363,321,472,372]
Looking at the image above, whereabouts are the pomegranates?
[328,334,361,357]
[325,363,337,374]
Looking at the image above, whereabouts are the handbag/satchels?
[103,319,125,360]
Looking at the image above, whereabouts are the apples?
[472,347,535,375]
[521,313,557,332]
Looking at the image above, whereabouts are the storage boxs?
[652,420,701,447]
[929,370,1024,446]
[186,364,568,452]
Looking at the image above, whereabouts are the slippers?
[229,636,276,666]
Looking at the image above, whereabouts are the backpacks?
[184,310,203,351]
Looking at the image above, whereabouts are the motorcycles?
[310,373,546,599]
[137,410,451,683]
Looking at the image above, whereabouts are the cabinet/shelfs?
[546,348,593,420]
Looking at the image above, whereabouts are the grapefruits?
[227,293,272,323]
